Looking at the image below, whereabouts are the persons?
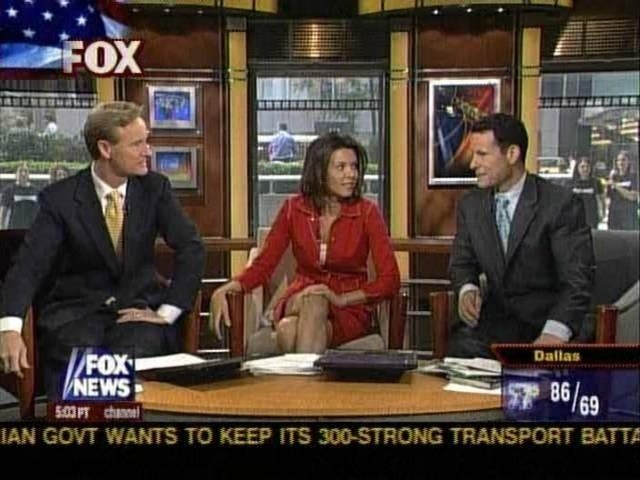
[2,101,208,380]
[8,118,36,134]
[208,132,403,353]
[444,113,600,360]
[265,122,298,161]
[47,164,72,181]
[42,113,58,136]
[606,150,638,230]
[1,164,39,230]
[568,156,605,230]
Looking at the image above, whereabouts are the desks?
[134,353,504,422]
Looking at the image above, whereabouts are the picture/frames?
[427,76,502,187]
[147,85,197,130]
[150,145,198,189]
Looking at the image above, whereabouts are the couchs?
[1,273,204,421]
[430,229,640,356]
[225,228,410,357]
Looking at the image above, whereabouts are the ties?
[496,197,510,252]
[104,191,124,255]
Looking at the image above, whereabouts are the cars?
[538,156,568,173]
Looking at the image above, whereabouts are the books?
[419,351,555,397]
[125,352,222,370]
[241,352,325,378]
